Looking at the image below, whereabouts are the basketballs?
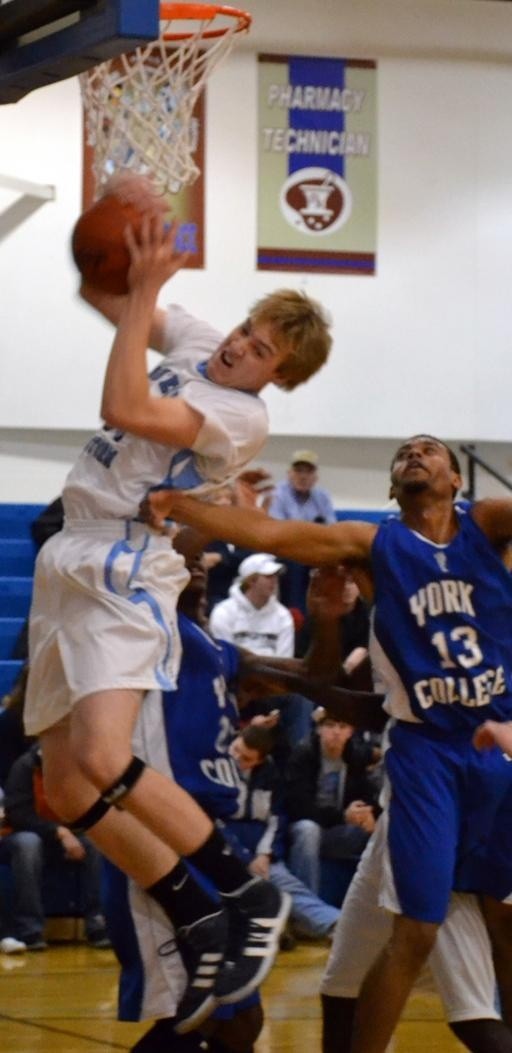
[71,195,156,296]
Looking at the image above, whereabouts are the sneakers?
[26,933,49,952]
[172,915,225,1034]
[87,930,111,950]
[1,938,25,954]
[213,878,292,1009]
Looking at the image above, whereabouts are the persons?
[0,661,36,792]
[228,725,340,952]
[269,447,334,525]
[143,432,512,1053]
[473,719,512,755]
[84,467,360,1053]
[5,740,112,953]
[283,718,377,895]
[25,212,333,1033]
[337,572,371,694]
[1,841,28,954]
[320,809,512,1052]
[210,553,315,767]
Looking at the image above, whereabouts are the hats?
[232,468,276,494]
[289,446,319,471]
[236,551,285,583]
